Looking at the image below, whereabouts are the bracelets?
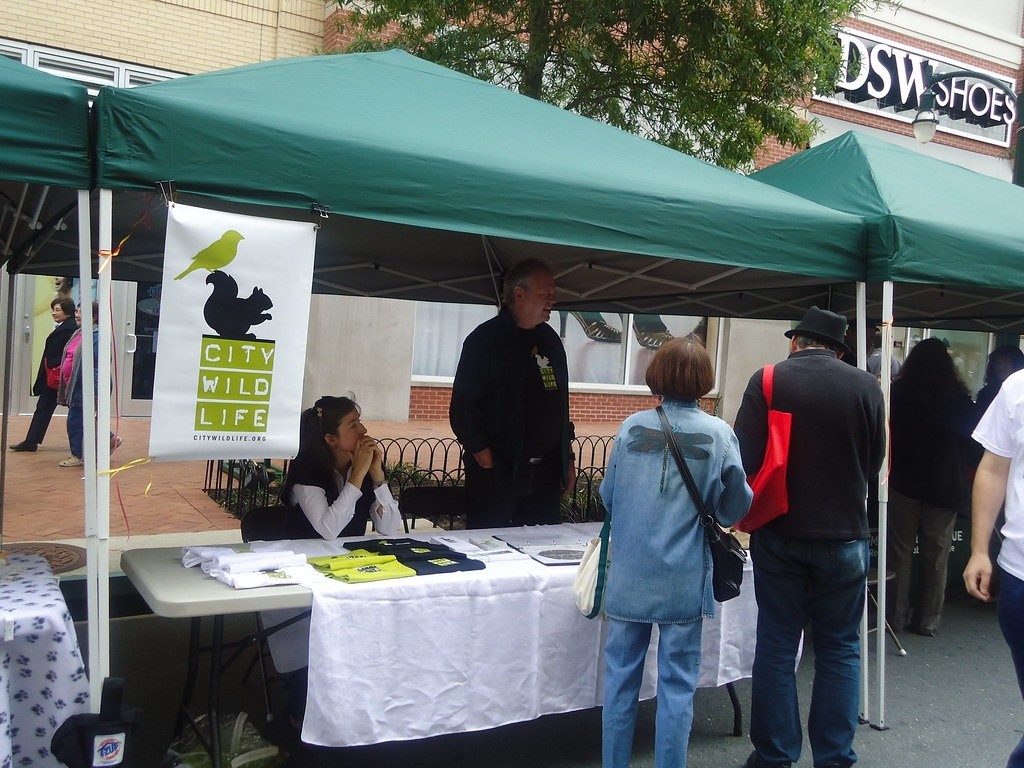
[373,481,388,489]
[568,452,576,461]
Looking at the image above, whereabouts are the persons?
[276,395,402,541]
[449,259,576,530]
[598,337,755,768]
[52,276,81,307]
[56,302,123,467]
[10,297,80,452]
[886,337,979,636]
[733,306,886,768]
[976,345,1024,602]
[963,369,1024,767]
[840,317,902,386]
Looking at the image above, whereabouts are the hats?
[784,306,850,355]
[849,317,880,332]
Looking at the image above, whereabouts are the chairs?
[222,506,313,723]
[398,486,472,530]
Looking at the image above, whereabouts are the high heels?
[560,309,620,343]
[633,321,677,348]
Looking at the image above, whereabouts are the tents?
[9,48,870,724]
[745,131,1024,730]
[0,55,99,714]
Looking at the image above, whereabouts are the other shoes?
[746,751,791,768]
[110,436,122,455]
[10,442,37,451]
[59,455,84,467]
[825,759,850,768]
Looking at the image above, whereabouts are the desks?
[118,521,803,760]
[0,554,91,768]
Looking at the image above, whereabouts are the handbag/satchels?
[734,364,793,533]
[48,367,60,389]
[575,512,612,620]
[710,523,747,602]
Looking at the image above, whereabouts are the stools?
[866,568,909,657]
